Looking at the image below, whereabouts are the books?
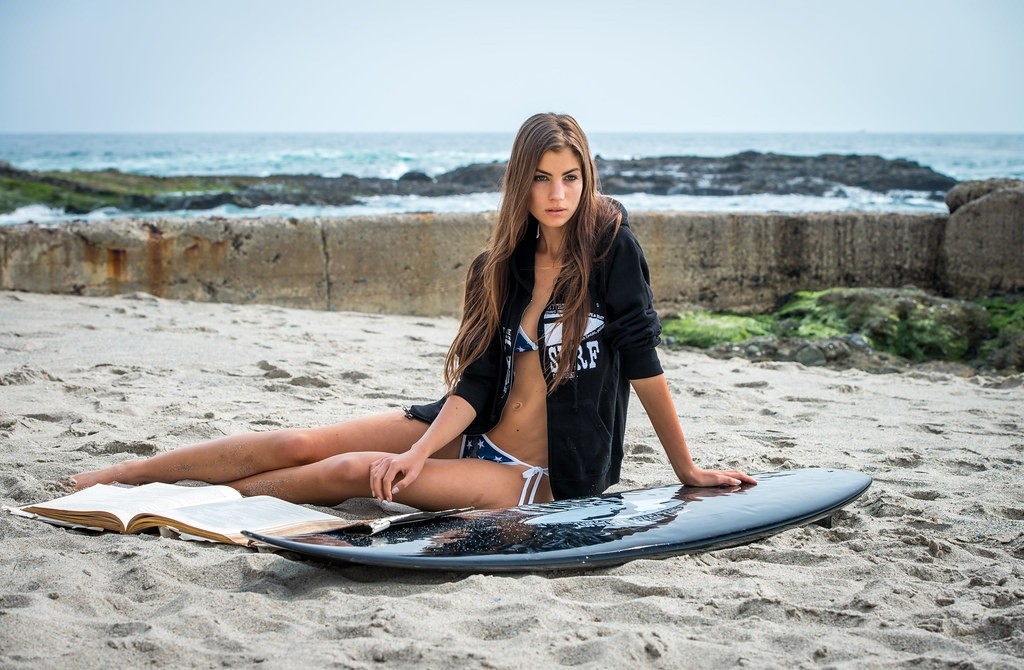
[7,482,350,548]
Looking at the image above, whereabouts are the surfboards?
[236,465,876,577]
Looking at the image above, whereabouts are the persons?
[67,112,757,512]
